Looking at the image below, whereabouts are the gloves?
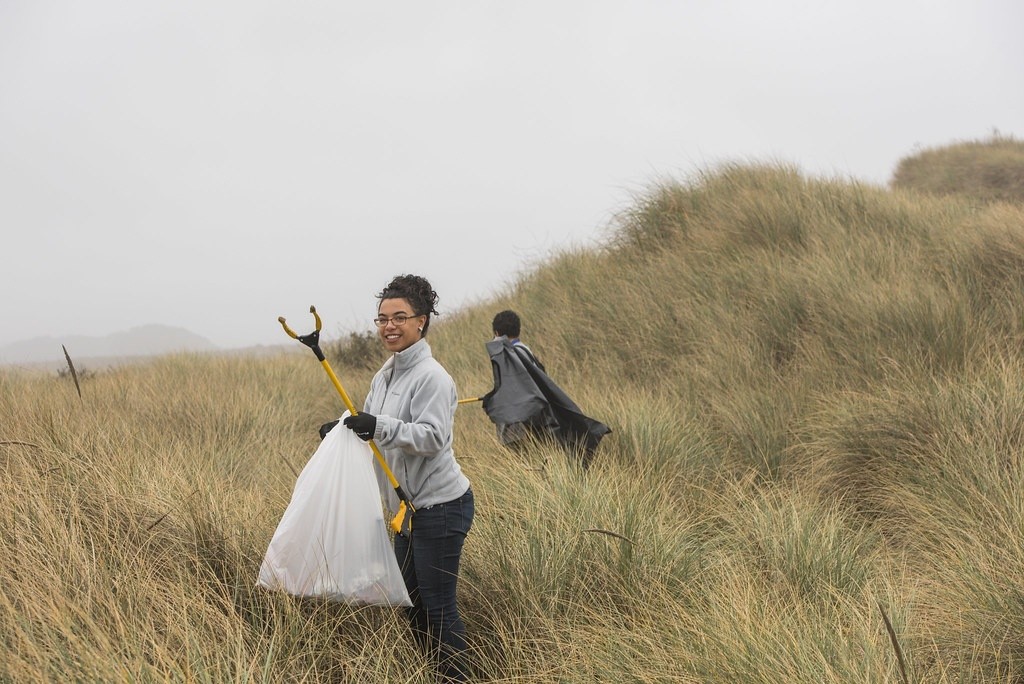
[344,412,376,441]
[319,421,339,440]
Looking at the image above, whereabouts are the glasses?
[374,315,418,327]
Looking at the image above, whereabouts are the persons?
[318,274,475,684]
[491,309,536,455]
